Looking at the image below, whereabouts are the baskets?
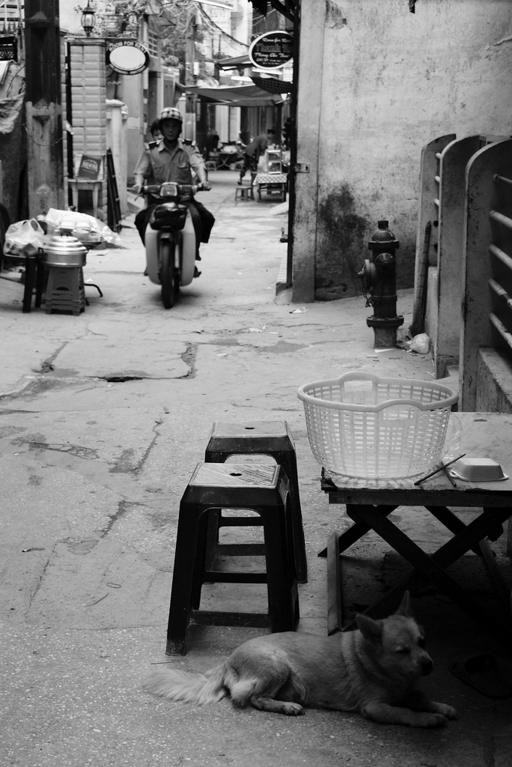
[298,373,457,480]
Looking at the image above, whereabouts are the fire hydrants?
[358,220,405,348]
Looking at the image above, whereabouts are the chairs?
[0,203,45,312]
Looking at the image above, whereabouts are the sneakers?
[196,241,202,260]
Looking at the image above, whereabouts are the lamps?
[81,0,96,36]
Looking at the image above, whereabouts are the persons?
[283,118,291,151]
[199,134,219,161]
[238,128,275,186]
[133,106,214,260]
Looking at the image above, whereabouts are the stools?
[45,267,85,315]
[235,186,254,201]
[236,162,245,169]
[165,420,307,656]
[205,161,216,170]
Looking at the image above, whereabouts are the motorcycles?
[134,180,211,308]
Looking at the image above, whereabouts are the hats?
[158,108,183,121]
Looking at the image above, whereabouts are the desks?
[69,179,103,219]
[317,412,512,635]
[252,173,288,201]
[210,152,238,170]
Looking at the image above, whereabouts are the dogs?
[135,587,461,731]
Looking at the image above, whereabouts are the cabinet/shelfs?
[266,149,282,195]
